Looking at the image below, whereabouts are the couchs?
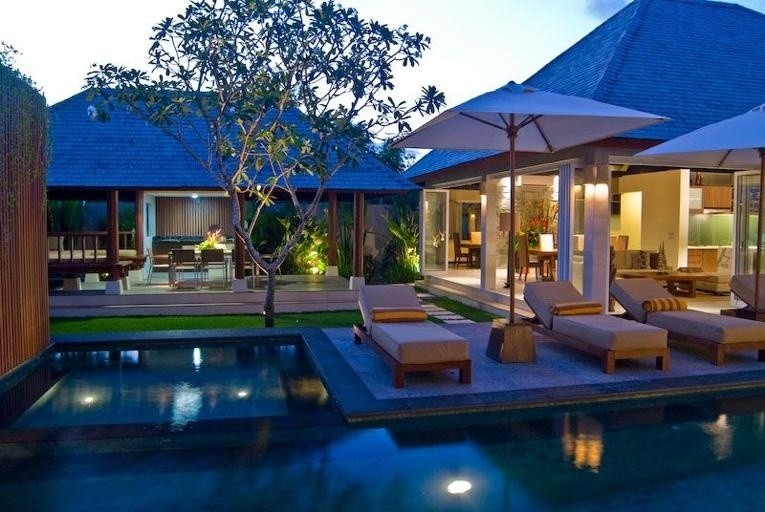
[522,279,672,374]
[720,274,765,322]
[354,283,472,388]
[614,249,672,274]
[677,267,733,295]
[609,278,765,365]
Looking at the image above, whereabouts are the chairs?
[182,246,204,282]
[471,231,481,267]
[146,248,176,285]
[573,235,586,252]
[519,234,542,282]
[615,234,629,250]
[170,250,197,290]
[539,233,557,281]
[200,249,231,289]
[452,233,473,268]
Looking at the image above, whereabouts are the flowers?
[519,198,561,233]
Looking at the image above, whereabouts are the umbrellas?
[634,104,765,309]
[387,81,671,321]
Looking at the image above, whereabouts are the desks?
[622,274,696,298]
[462,243,482,268]
[530,250,583,281]
[169,249,233,287]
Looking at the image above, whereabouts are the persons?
[203,223,223,243]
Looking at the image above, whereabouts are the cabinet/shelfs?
[688,246,733,273]
